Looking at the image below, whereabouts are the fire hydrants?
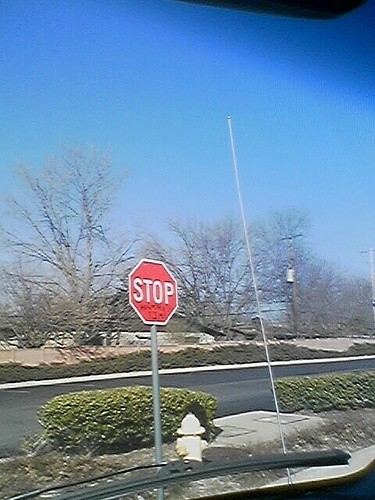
[176,413,208,462]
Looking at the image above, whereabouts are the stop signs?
[129,259,177,325]
